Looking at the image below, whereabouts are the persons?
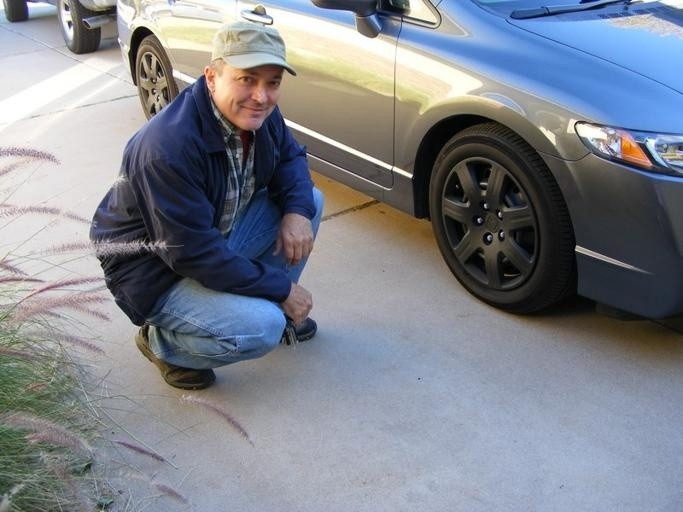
[87,26,327,395]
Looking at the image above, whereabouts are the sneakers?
[278,316,318,342]
[134,324,216,389]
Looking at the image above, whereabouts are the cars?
[1,0,115,55]
[115,0,682,326]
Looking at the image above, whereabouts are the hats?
[211,23,297,76]
